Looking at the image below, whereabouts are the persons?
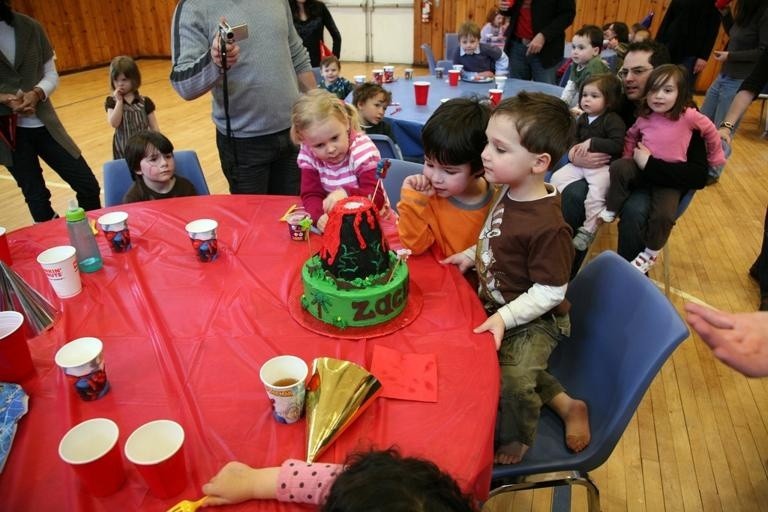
[120,130,197,203]
[202,436,479,511]
[104,55,160,160]
[0,0,101,223]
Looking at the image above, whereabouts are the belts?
[514,36,532,48]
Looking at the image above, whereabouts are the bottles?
[65,198,103,274]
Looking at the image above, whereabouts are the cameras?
[218,21,248,44]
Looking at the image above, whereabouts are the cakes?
[298,196,412,330]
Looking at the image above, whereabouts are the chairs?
[471,248,691,511]
[380,156,423,220]
[544,132,733,309]
[101,148,210,211]
[310,66,323,84]
[420,32,462,77]
[365,133,401,161]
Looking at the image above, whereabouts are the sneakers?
[597,206,617,223]
[630,250,658,274]
[573,228,593,251]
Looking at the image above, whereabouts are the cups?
[285,211,310,242]
[184,218,222,263]
[259,354,308,426]
[97,211,133,255]
[53,335,111,400]
[122,418,189,499]
[0,309,38,382]
[57,416,127,499]
[0,226,11,268]
[36,244,84,300]
[353,63,507,106]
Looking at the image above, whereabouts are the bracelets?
[33,87,45,100]
